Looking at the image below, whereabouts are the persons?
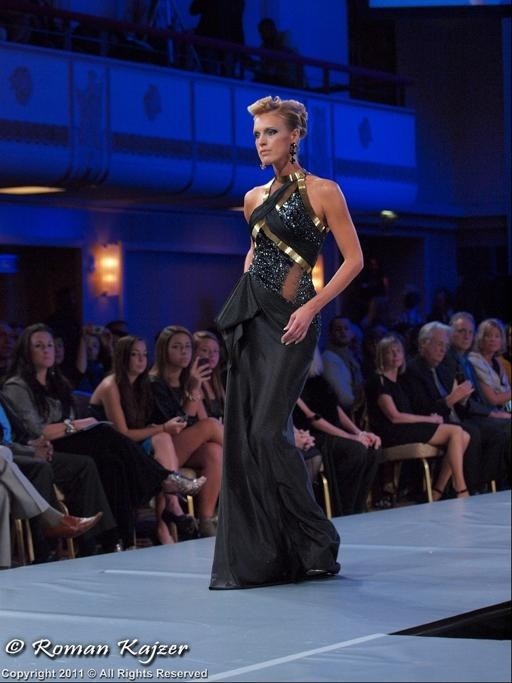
[187,0,247,77]
[339,255,458,310]
[208,92,368,590]
[248,17,307,89]
[1,310,512,571]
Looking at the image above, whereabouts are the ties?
[430,367,461,423]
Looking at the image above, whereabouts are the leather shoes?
[41,512,104,542]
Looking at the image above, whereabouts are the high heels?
[162,473,208,503]
[160,509,200,539]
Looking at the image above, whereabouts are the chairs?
[12,441,496,568]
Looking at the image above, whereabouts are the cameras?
[92,325,104,335]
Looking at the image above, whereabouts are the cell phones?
[198,357,212,379]
[455,372,465,385]
[177,414,188,422]
